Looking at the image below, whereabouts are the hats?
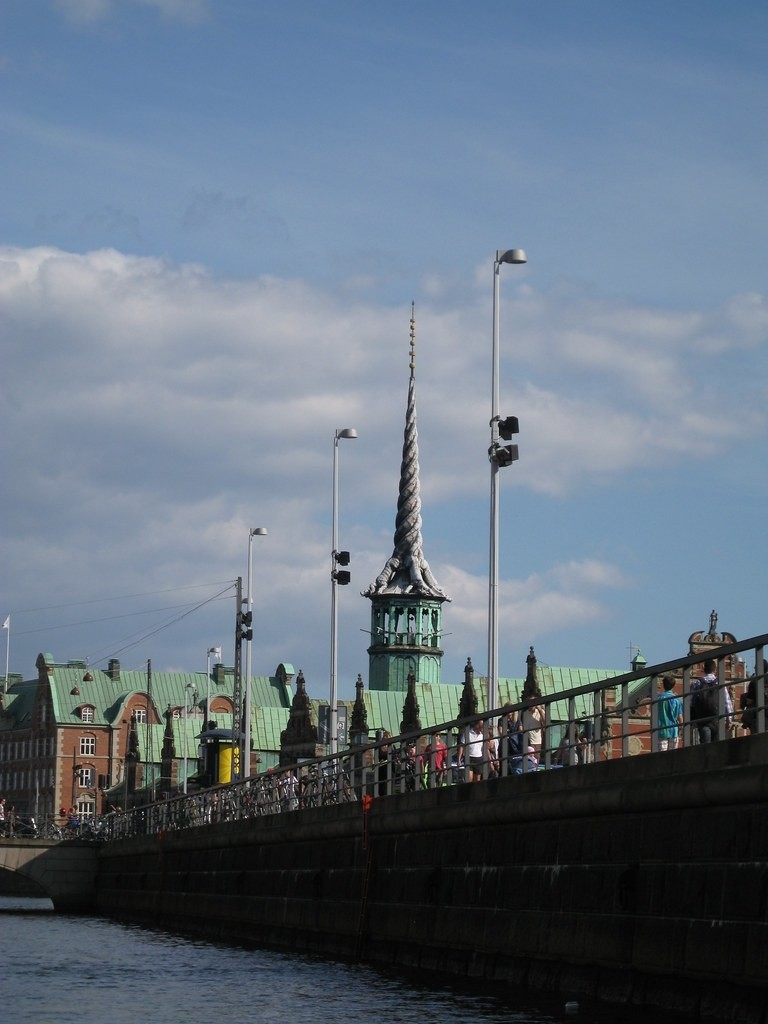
[59,812,62,814]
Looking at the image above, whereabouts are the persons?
[0,797,63,839]
[656,657,735,751]
[61,767,357,839]
[552,724,587,767]
[743,657,768,735]
[379,695,546,796]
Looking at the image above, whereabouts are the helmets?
[59,807,66,814]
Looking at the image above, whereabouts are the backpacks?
[690,677,718,721]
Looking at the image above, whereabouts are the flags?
[2,614,10,628]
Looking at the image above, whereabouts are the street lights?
[180,682,196,793]
[245,527,266,786]
[486,244,529,736]
[328,429,358,772]
[207,647,220,721]
